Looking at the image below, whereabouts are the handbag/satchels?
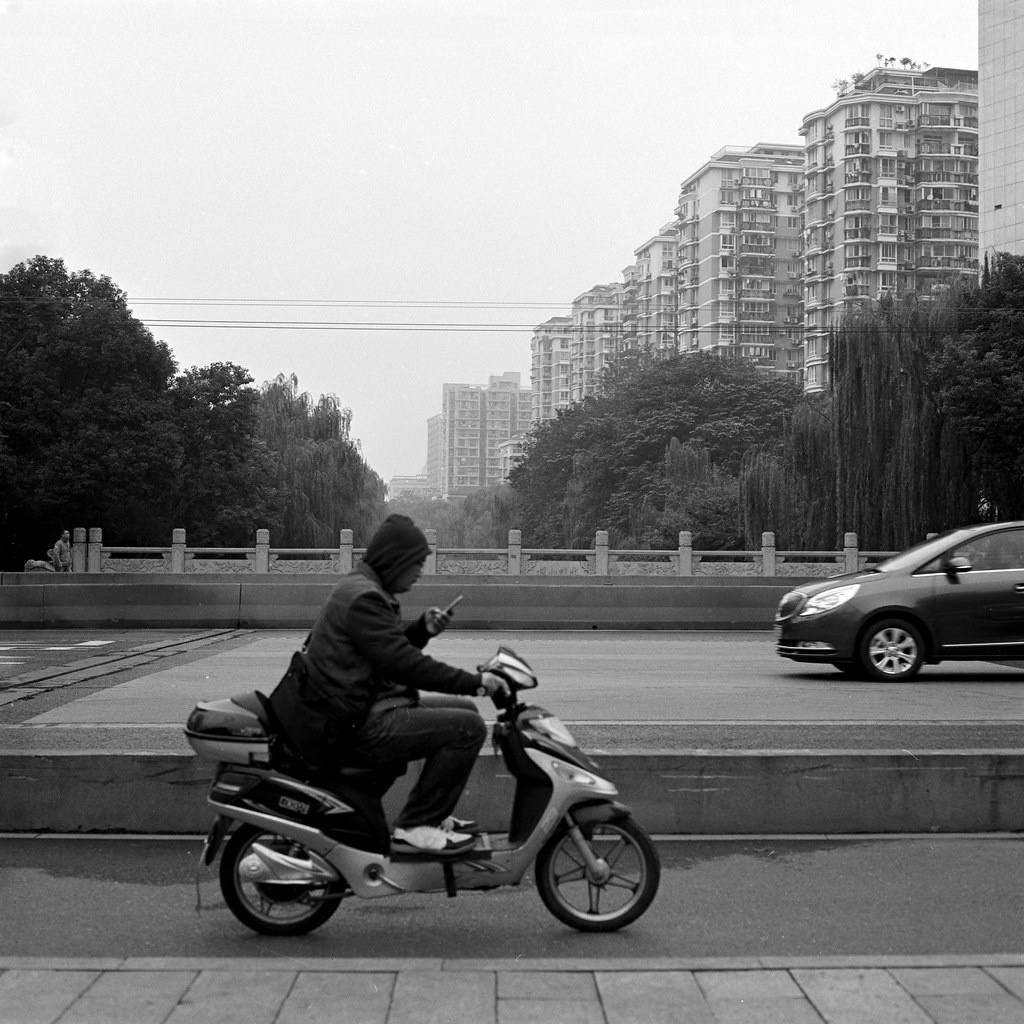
[264,652,361,773]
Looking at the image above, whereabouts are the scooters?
[184,644,661,938]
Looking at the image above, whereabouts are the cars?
[771,520,1024,681]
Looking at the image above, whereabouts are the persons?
[52,530,73,573]
[967,542,1021,568]
[266,514,511,855]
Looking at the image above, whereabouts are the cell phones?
[443,595,465,613]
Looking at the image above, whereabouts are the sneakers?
[391,822,476,855]
[441,815,482,834]
